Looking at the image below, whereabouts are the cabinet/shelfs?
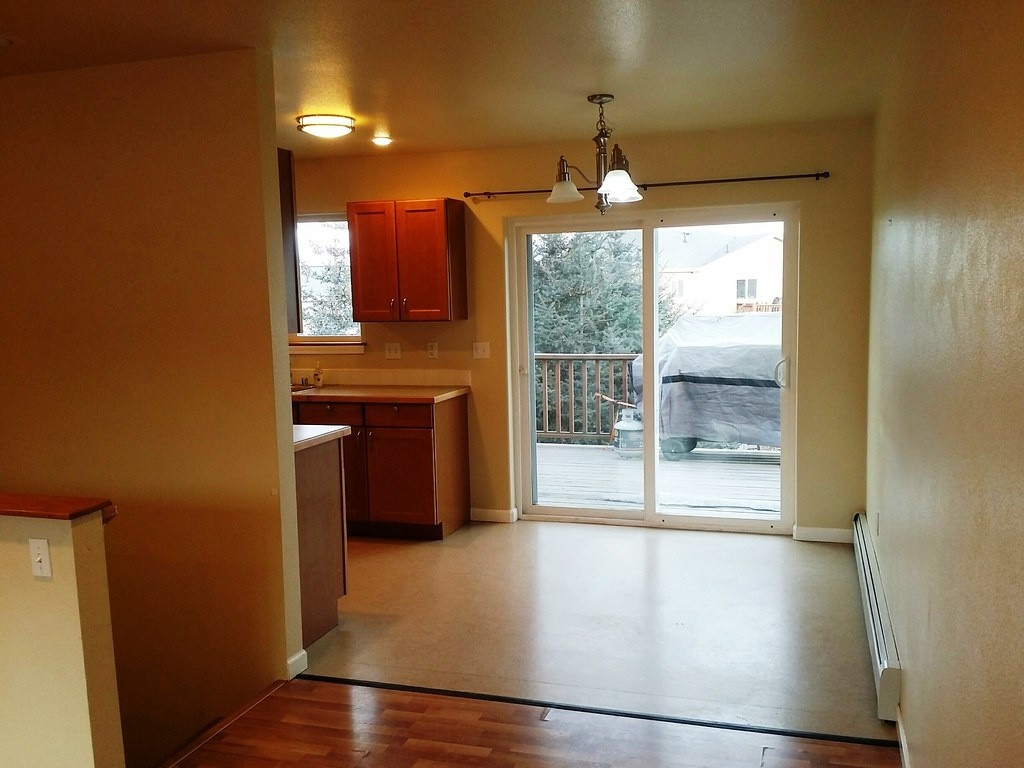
[345,197,468,322]
[292,383,471,540]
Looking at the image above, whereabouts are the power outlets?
[472,341,489,359]
[385,342,401,360]
[427,342,438,358]
[29,539,52,577]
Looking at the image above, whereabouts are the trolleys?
[659,315,786,463]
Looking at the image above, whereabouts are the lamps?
[372,137,395,146]
[295,113,356,138]
[546,93,645,216]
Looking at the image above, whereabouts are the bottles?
[313,361,323,388]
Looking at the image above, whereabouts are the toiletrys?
[312,360,324,389]
